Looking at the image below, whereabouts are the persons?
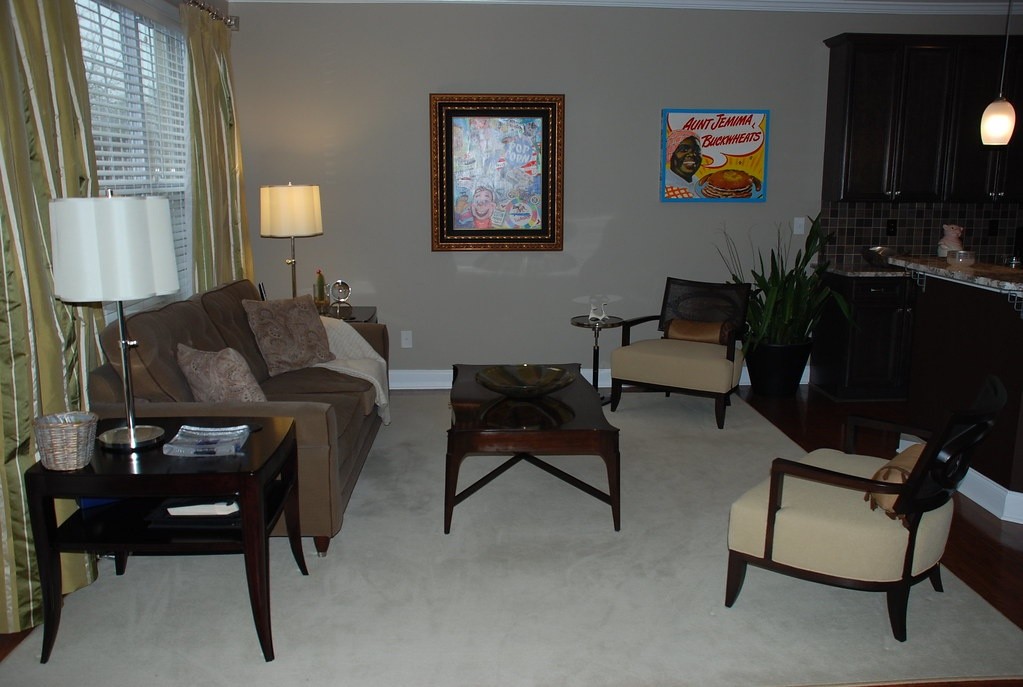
[665,130,702,199]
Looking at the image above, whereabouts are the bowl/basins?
[946,251,975,267]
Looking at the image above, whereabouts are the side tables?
[21,415,309,667]
[318,306,378,323]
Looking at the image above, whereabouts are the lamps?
[46,192,180,450]
[978,0,1019,146]
[257,180,325,299]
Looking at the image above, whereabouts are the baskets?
[31,411,99,471]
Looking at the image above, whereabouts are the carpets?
[0,388,1023,687]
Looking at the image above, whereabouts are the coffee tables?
[442,364,618,539]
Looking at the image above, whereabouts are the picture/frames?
[660,108,770,203]
[428,89,566,253]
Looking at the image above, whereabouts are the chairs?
[721,409,1000,644]
[610,276,753,431]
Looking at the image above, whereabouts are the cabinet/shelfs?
[948,34,1023,205]
[809,272,919,402]
[820,31,957,204]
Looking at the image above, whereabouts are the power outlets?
[400,330,412,349]
[886,219,898,237]
[989,219,999,236]
[792,216,806,236]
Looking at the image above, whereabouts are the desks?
[569,314,624,407]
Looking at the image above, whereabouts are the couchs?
[87,278,391,562]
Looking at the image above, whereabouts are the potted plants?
[709,209,864,399]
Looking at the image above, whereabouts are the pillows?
[174,341,268,403]
[240,294,337,377]
